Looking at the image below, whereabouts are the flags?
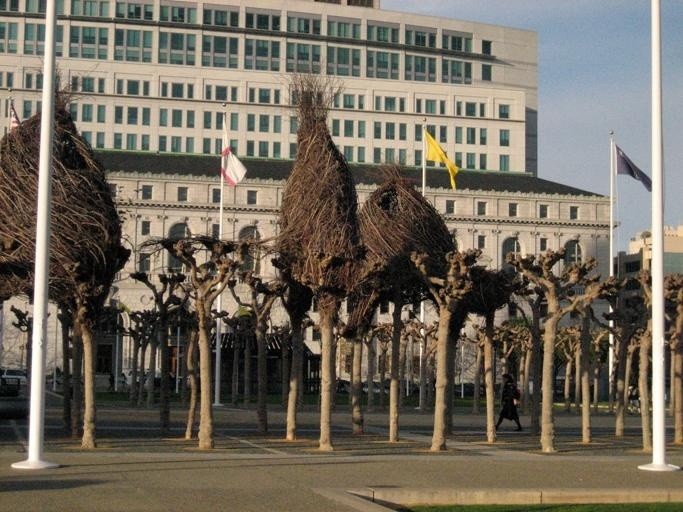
[118,299,130,314]
[221,116,247,187]
[615,144,652,193]
[426,129,459,192]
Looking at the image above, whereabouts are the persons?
[494,374,523,431]
[628,386,640,413]
[120,373,128,393]
[108,373,115,391]
